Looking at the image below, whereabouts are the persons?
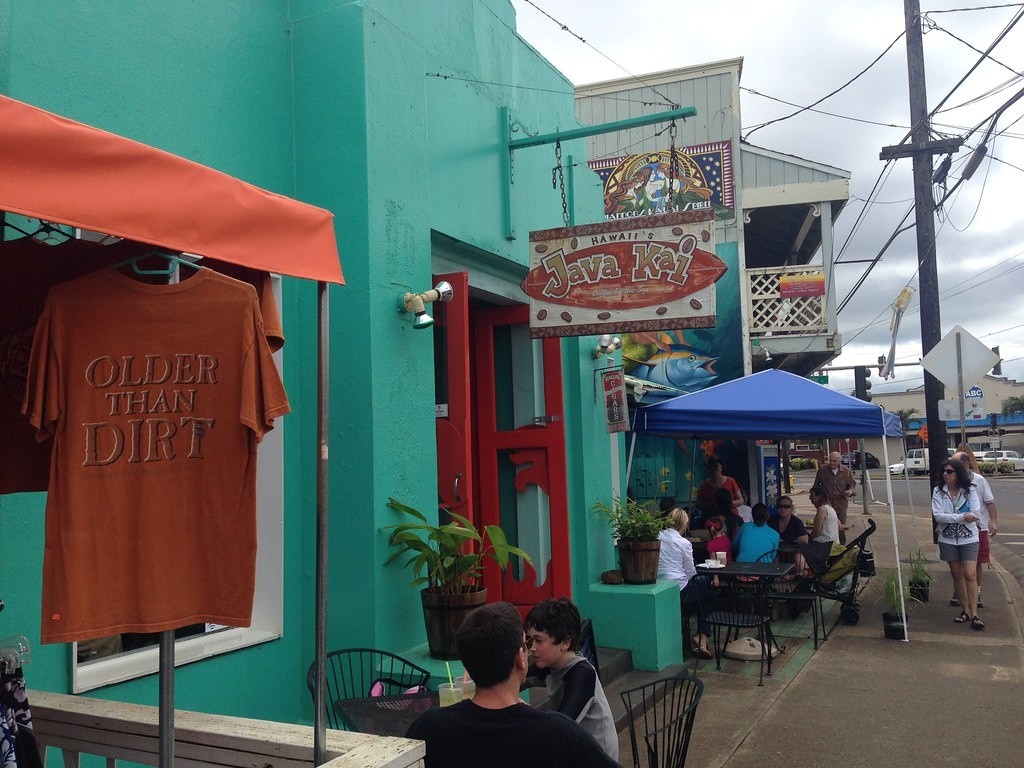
[657,456,843,659]
[518,596,619,763]
[404,601,623,768]
[931,442,997,627]
[813,452,857,543]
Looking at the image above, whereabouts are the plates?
[698,563,725,569]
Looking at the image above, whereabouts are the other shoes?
[950,592,961,606]
[977,594,984,607]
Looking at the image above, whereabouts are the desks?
[777,542,799,563]
[697,561,797,664]
[334,691,438,737]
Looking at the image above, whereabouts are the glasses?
[520,635,533,649]
[777,504,792,509]
[942,468,955,474]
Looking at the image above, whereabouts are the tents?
[0,95,346,768]
[627,368,920,642]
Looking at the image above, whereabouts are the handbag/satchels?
[859,550,876,577]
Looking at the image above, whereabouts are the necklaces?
[950,492,956,498]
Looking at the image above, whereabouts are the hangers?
[32,220,200,275]
[0,636,32,674]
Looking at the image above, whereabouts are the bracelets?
[850,491,853,494]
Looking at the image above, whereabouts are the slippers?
[692,647,713,660]
[955,612,970,622]
[971,616,985,629]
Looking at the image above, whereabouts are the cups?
[438,682,463,707]
[716,551,727,566]
[705,559,720,566]
[453,676,476,700]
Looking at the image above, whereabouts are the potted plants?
[377,495,536,661]
[869,564,926,641]
[903,549,936,602]
[593,487,677,584]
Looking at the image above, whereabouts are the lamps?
[410,293,436,330]
[606,336,623,355]
[597,334,613,348]
[765,348,773,363]
[754,339,769,350]
[423,281,454,305]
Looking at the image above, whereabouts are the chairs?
[620,535,828,768]
[306,648,433,731]
